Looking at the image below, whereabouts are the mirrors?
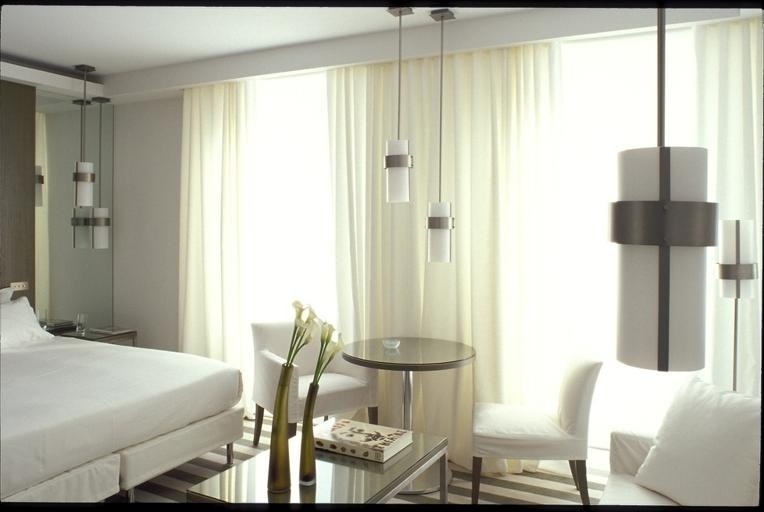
[37,90,116,327]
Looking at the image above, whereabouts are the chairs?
[249,321,381,447]
[469,358,601,507]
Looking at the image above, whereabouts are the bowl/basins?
[382,338,400,350]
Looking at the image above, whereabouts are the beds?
[0,335,245,506]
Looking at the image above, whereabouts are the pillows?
[0,281,52,348]
[633,375,762,509]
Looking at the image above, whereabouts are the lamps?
[381,5,459,267]
[72,63,112,255]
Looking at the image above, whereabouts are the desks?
[58,325,139,346]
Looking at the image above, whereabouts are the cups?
[74,312,87,336]
[37,307,47,330]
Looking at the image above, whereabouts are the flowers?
[285,300,348,382]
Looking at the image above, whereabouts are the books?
[88,325,136,337]
[311,417,414,464]
[311,441,415,475]
